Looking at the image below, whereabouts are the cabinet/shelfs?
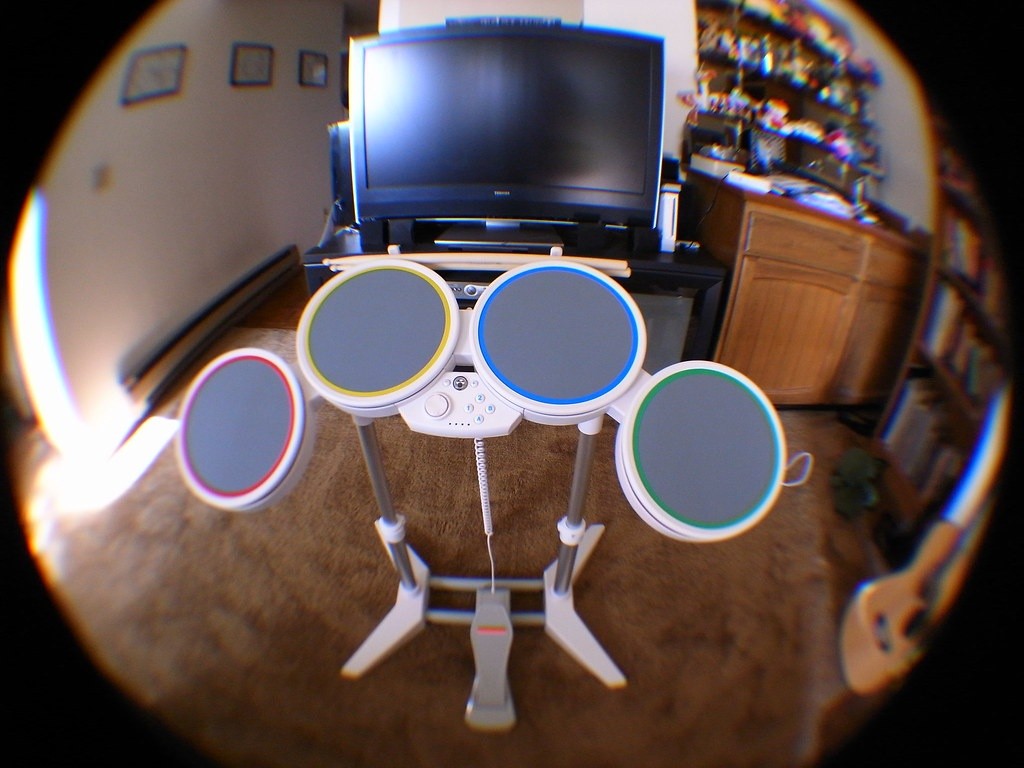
[683,164,930,408]
[867,123,1005,563]
[680,0,883,197]
[304,225,730,372]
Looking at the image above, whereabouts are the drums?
[294,258,461,418]
[468,260,649,426]
[615,360,789,544]
[174,346,316,514]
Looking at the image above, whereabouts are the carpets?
[38,326,834,767]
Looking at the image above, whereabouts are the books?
[946,217,1006,313]
[925,283,995,395]
[882,378,971,507]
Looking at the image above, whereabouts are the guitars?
[838,500,994,697]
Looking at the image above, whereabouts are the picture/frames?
[123,43,186,104]
[298,50,329,87]
[230,43,273,86]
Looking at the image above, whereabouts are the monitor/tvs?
[348,22,665,248]
